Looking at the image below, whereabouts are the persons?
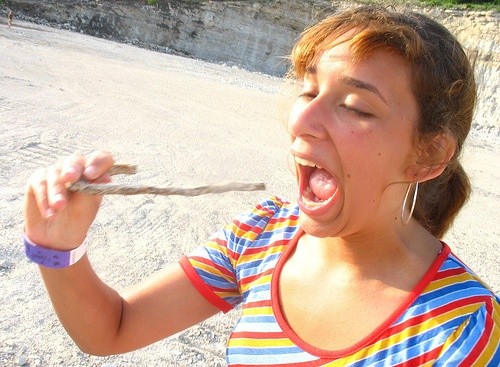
[21,5,500,367]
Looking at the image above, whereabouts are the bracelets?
[24,234,87,269]
